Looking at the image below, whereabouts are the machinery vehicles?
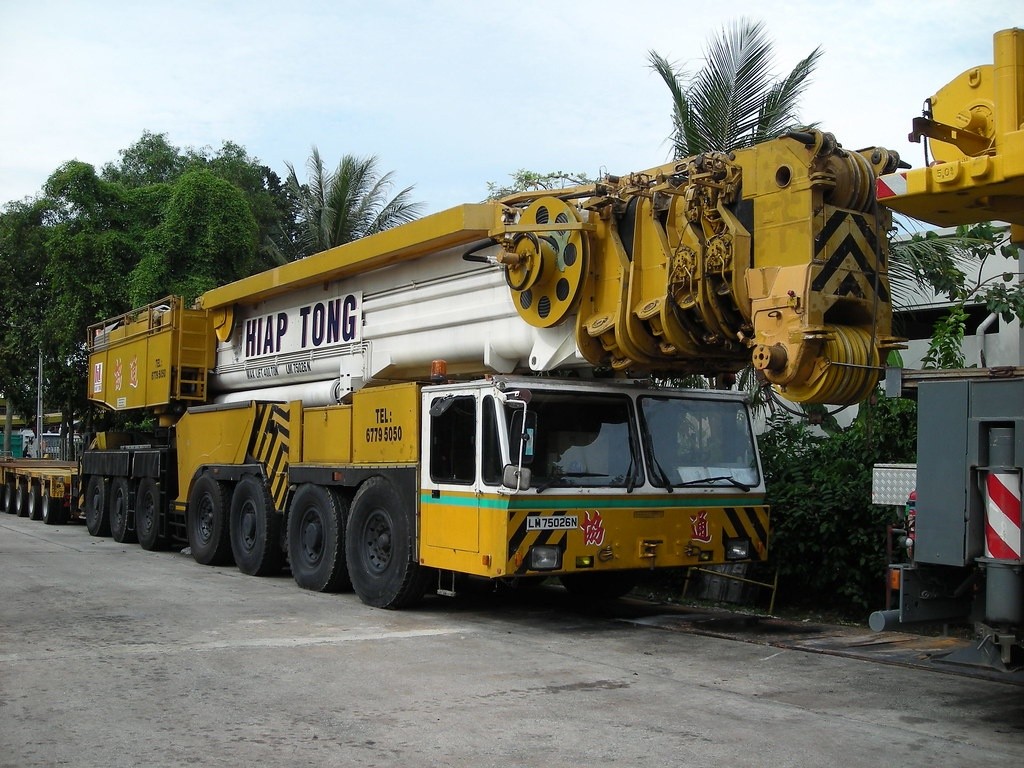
[868,26,1024,663]
[75,129,891,607]
